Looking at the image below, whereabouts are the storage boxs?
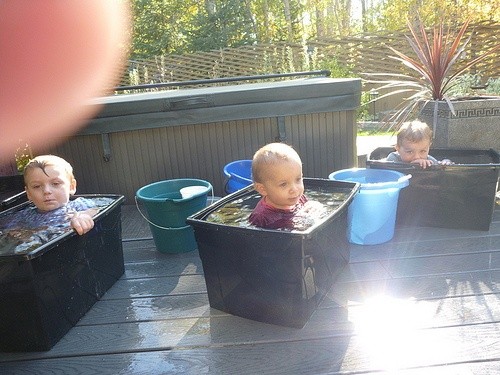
[366,145,500,232]
[185,177,361,329]
[0,194,127,353]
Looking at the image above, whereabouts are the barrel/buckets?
[327,167,410,246]
[136,177,214,255]
[223,159,255,195]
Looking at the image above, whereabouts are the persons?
[384,120,454,169]
[249,144,308,229]
[6,154,99,236]
